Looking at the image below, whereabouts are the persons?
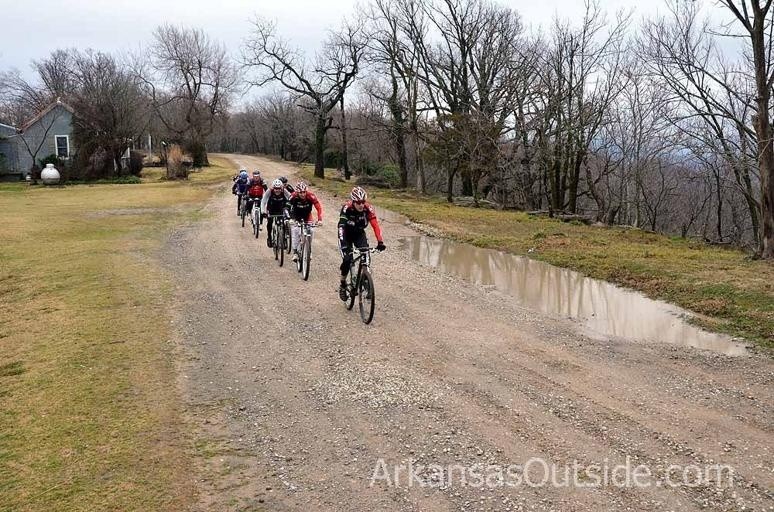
[338,187,386,301]
[232,168,323,261]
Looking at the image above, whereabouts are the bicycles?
[344,247,380,324]
[234,191,320,281]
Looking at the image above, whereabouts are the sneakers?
[259,223,263,230]
[237,209,240,216]
[363,281,369,289]
[292,253,299,263]
[339,281,348,301]
[267,236,272,248]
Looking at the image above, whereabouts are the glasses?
[356,200,366,205]
[275,188,281,190]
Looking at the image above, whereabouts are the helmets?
[350,186,367,201]
[272,179,283,187]
[295,181,308,193]
[252,170,261,177]
[279,176,288,183]
[240,168,248,179]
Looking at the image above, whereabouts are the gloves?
[377,241,386,252]
[232,191,237,195]
[289,218,296,225]
[246,196,250,201]
[341,247,352,253]
[262,213,268,218]
[318,220,323,226]
